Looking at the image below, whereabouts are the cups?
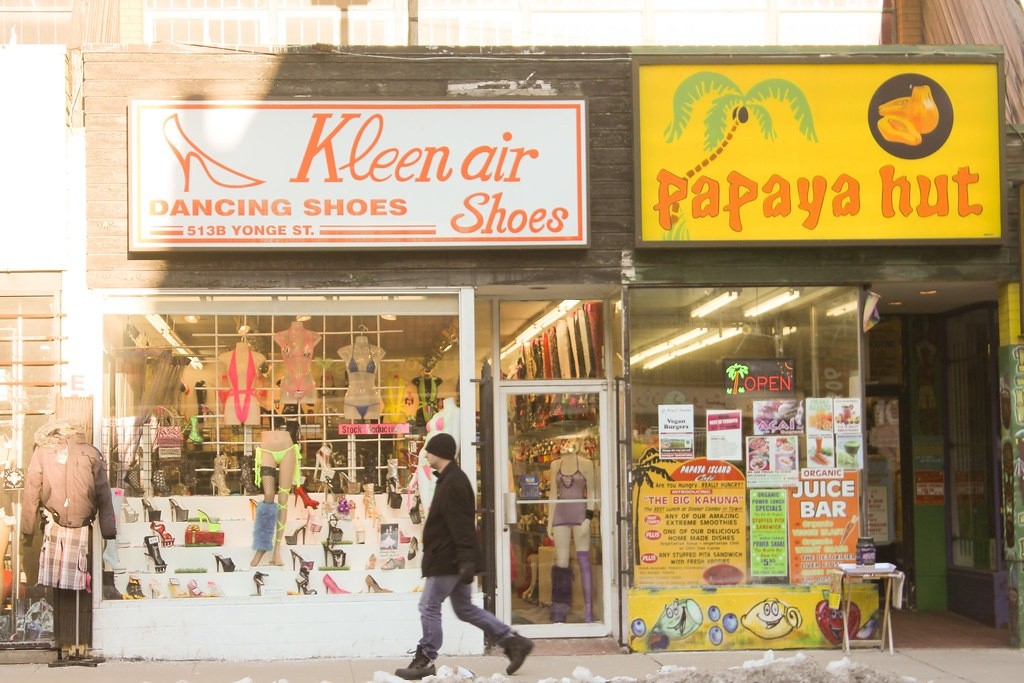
[841,406,851,418]
[816,408,825,420]
[750,458,769,471]
[815,437,822,454]
[631,434,649,460]
[844,442,860,460]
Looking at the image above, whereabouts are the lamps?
[296,315,311,322]
[380,314,397,321]
[235,323,250,335]
[145,316,204,370]
[627,287,801,371]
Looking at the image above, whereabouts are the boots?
[504,632,534,674]
[395,642,438,680]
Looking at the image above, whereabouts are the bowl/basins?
[778,404,791,417]
[778,456,793,471]
[780,445,794,456]
[749,438,768,450]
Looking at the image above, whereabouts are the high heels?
[102,417,420,599]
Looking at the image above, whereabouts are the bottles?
[856,537,876,568]
[650,427,658,448]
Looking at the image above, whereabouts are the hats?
[425,433,456,460]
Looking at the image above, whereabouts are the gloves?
[460,560,474,584]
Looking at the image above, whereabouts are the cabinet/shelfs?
[105,494,426,598]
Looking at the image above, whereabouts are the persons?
[337,336,386,419]
[218,342,266,425]
[250,431,296,566]
[20,422,118,590]
[395,433,535,680]
[407,397,460,522]
[273,321,321,404]
[411,372,443,428]
[383,374,410,424]
[546,451,595,623]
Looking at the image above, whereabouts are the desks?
[840,570,903,656]
